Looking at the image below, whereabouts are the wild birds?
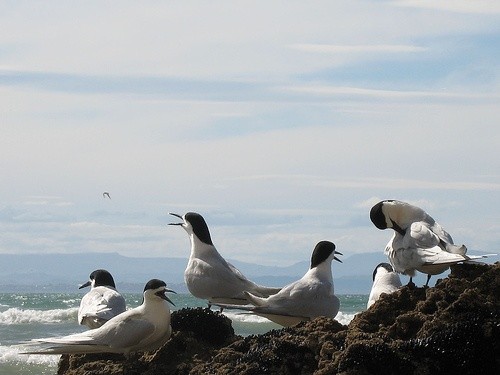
[17,278,177,369]
[78,268,126,330]
[102,192,111,200]
[206,241,343,329]
[167,212,282,318]
[367,262,402,312]
[369,200,498,290]
